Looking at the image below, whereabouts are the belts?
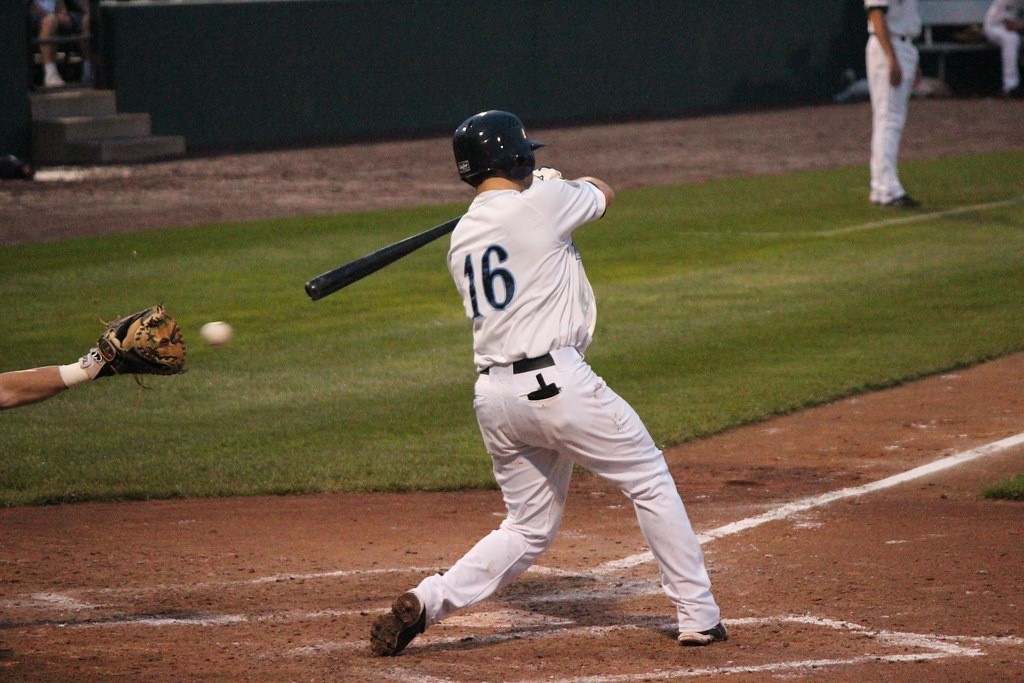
[480,347,580,375]
[901,36,906,41]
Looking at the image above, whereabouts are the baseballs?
[200,320,234,347]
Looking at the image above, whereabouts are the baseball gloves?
[89,301,188,390]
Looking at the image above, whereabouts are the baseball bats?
[302,216,461,301]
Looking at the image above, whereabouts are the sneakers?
[678,622,728,645]
[370,592,426,657]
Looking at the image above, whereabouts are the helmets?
[453,110,545,179]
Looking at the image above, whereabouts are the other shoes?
[46,74,65,87]
[888,195,922,209]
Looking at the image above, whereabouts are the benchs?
[917,0,994,98]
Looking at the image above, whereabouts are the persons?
[0,303,185,413]
[863,0,922,207]
[369,110,728,656]
[982,0,1024,97]
[32,0,89,85]
